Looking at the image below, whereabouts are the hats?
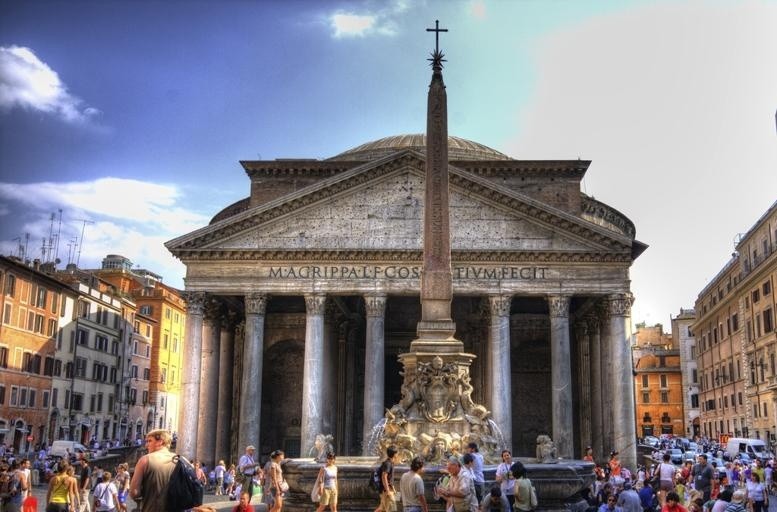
[245,443,256,451]
[119,462,130,471]
[643,477,654,484]
[270,449,283,458]
[733,491,744,504]
[217,460,235,469]
[584,445,594,451]
[609,450,619,456]
[462,442,479,453]
[11,459,24,467]
[80,456,88,462]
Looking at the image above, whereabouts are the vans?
[725,436,774,464]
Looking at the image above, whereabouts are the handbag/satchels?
[390,490,401,503]
[259,472,266,486]
[23,496,38,512]
[234,473,246,484]
[270,481,289,496]
[529,487,539,508]
[94,499,101,508]
[655,476,660,491]
[310,479,324,503]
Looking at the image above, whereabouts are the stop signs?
[27,436,33,441]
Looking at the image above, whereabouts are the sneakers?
[208,489,234,497]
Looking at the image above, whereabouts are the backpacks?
[1,469,19,498]
[167,455,203,512]
[369,461,392,492]
[432,473,450,502]
[237,455,248,472]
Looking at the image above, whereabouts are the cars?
[643,434,700,465]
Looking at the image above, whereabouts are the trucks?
[50,441,89,459]
